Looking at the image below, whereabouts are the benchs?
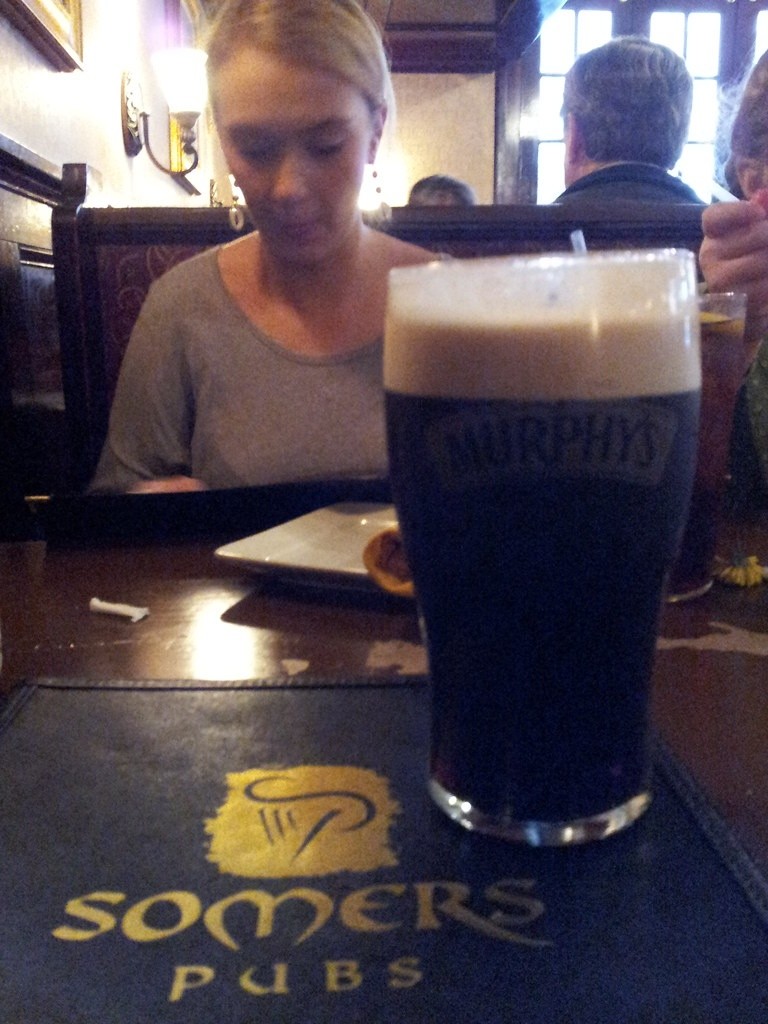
[54,205,712,478]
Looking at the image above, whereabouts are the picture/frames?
[165,0,214,196]
[0,0,87,73]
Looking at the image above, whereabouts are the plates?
[216,500,399,596]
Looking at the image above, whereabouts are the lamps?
[119,47,211,176]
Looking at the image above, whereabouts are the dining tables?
[2,515,768,1023]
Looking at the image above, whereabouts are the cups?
[666,287,746,605]
[384,249,701,846]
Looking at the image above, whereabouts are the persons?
[549,37,705,205]
[689,45,768,513]
[87,0,464,493]
[405,175,474,206]
